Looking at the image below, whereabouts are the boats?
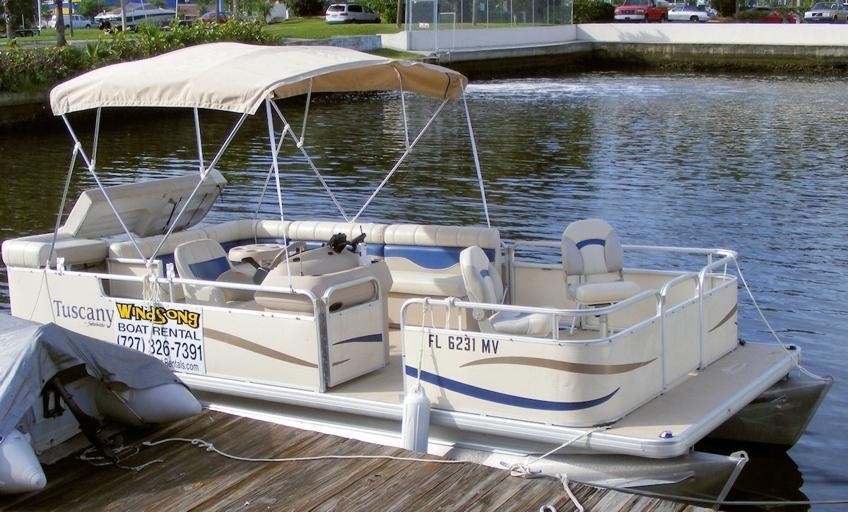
[1,41,802,458]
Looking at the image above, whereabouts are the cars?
[614,0,848,23]
[200,12,234,24]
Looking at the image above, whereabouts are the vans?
[326,3,381,24]
[51,15,93,29]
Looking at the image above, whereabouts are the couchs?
[105,218,501,330]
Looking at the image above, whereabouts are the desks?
[228,244,284,268]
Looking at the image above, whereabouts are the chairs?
[175,238,262,310]
[459,245,551,339]
[560,219,639,338]
